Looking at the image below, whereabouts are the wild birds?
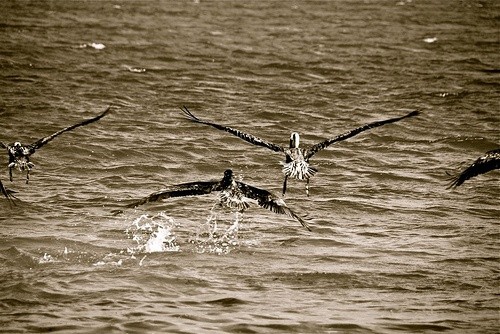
[443,151,500,191]
[110,170,314,234]
[177,103,423,197]
[0,104,112,183]
